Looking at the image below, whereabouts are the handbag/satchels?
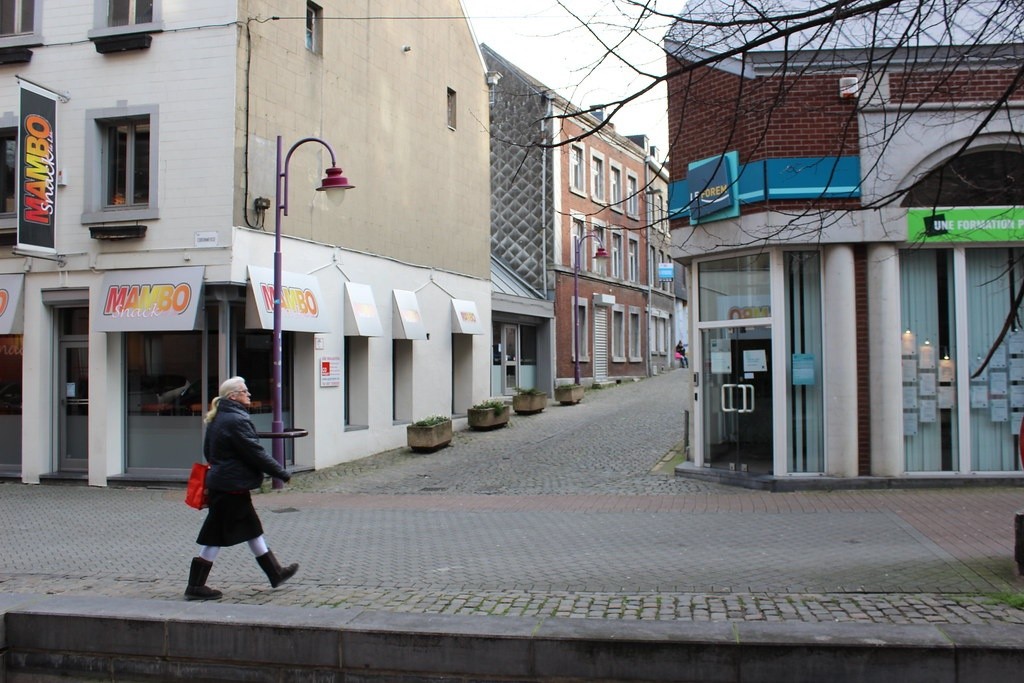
[185,462,211,510]
[675,352,681,358]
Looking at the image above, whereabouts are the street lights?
[271,133,355,492]
[573,234,611,385]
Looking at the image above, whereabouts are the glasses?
[234,388,249,394]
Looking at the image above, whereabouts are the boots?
[256,547,299,588]
[183,557,223,601]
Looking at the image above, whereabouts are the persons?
[184,376,299,600]
[676,340,689,368]
